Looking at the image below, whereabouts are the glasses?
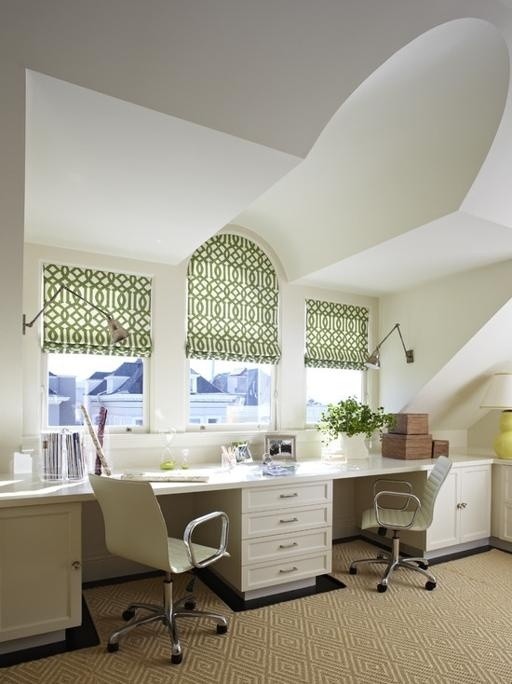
[23,285,130,344]
[364,323,415,370]
[479,372,512,459]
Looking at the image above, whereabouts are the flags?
[336,431,369,460]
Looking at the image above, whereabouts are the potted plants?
[265,435,296,460]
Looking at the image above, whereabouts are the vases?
[312,396,398,447]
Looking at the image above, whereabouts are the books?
[381,433,433,460]
[388,412,429,436]
[431,440,449,459]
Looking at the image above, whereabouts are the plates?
[0,453,512,654]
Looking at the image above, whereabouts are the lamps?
[350,456,454,593]
[88,473,232,665]
